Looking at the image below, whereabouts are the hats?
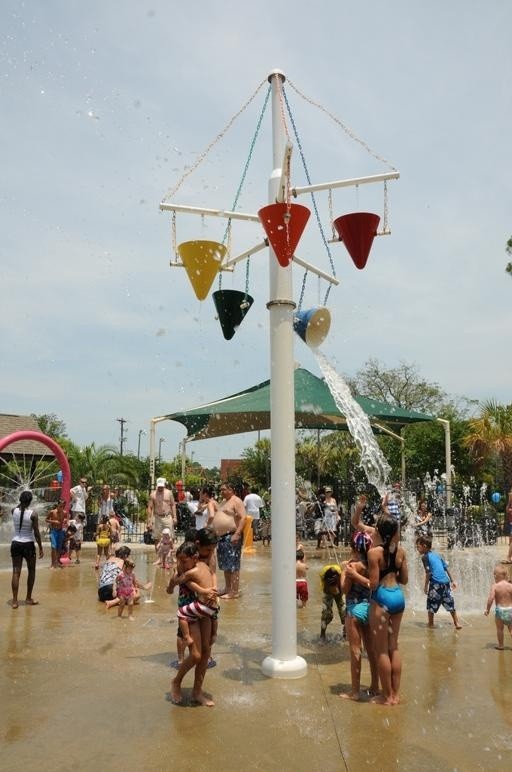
[162,528,171,537]
[325,487,332,492]
[352,531,373,551]
[157,478,166,487]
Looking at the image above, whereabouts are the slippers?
[206,657,216,668]
[171,661,183,669]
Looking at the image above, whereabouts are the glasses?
[80,481,87,483]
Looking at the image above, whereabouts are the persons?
[337,527,381,703]
[8,489,45,611]
[45,477,141,570]
[98,546,152,619]
[168,524,221,710]
[381,481,510,545]
[484,564,511,650]
[164,540,221,648]
[296,483,347,646]
[341,511,410,708]
[146,477,271,599]
[417,536,462,629]
